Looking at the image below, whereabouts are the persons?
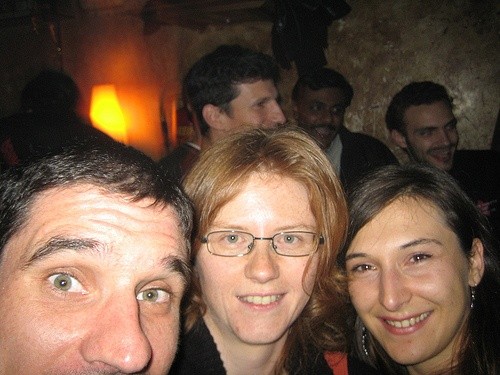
[157,44,286,188]
[336,162,499,375]
[292,68,400,190]
[167,125,390,375]
[386,80,500,203]
[0,136,193,375]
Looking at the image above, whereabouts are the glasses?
[200,231,325,258]
